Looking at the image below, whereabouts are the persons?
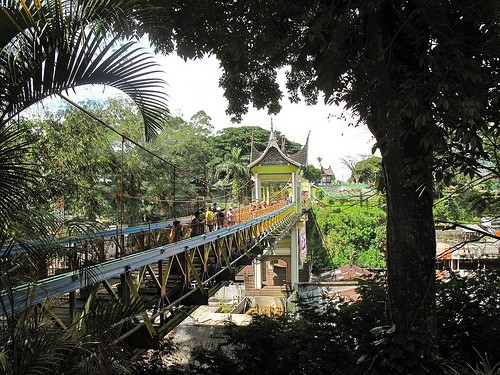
[205,205,216,232]
[171,220,186,274]
[198,207,207,235]
[216,208,237,230]
[212,203,219,230]
[283,191,309,206]
[249,198,283,219]
[189,212,206,262]
